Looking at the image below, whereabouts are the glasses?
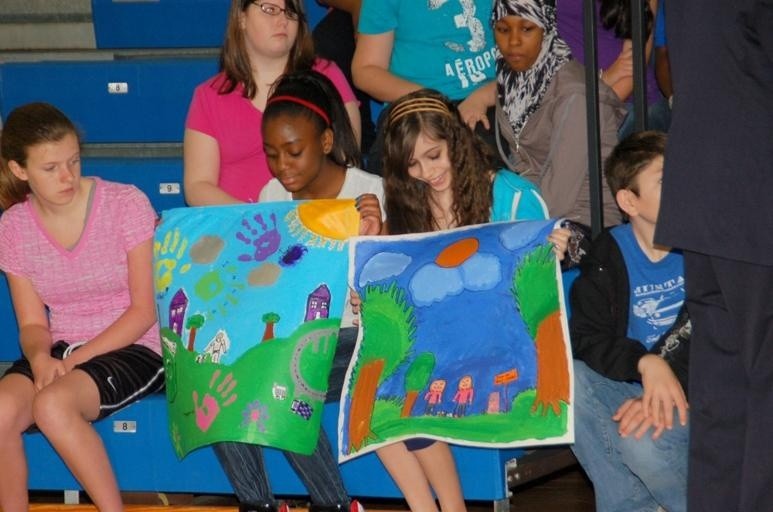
[253,0,299,22]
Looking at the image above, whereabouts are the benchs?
[0,270,582,512]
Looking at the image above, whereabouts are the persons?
[556,0,659,138]
[183,1,361,209]
[206,70,387,512]
[0,102,167,512]
[350,0,508,182]
[567,128,688,512]
[311,1,385,154]
[645,1,675,130]
[489,0,629,270]
[653,0,773,511]
[348,88,552,512]
